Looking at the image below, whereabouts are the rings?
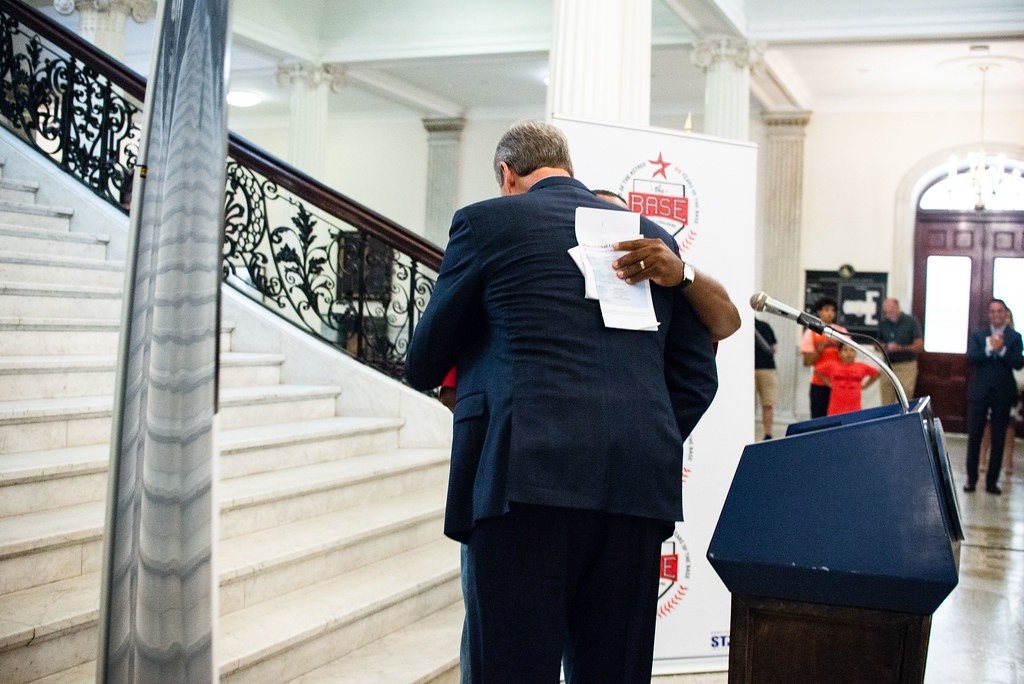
[640,261,646,271]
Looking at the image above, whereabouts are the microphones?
[748,291,826,327]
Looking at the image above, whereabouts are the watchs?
[674,261,695,292]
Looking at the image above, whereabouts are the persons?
[592,189,741,357]
[754,319,778,442]
[802,299,1024,497]
[402,122,720,684]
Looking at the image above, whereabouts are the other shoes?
[985,482,1002,495]
[963,474,979,491]
